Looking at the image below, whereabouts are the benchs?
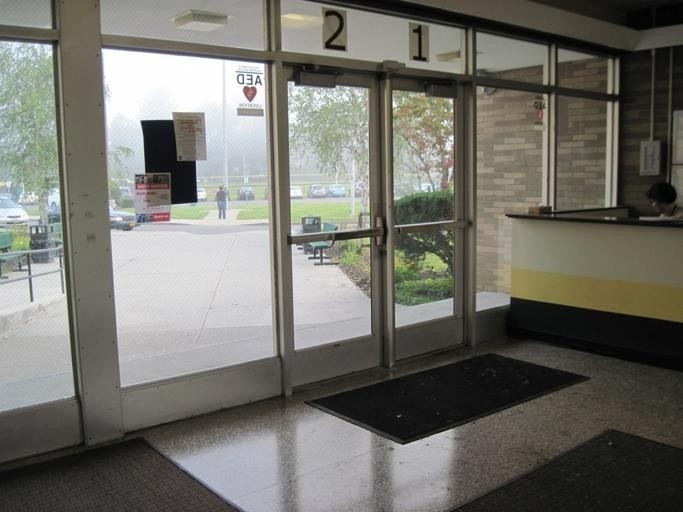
[0,231,28,279]
[309,222,340,265]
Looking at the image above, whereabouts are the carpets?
[305,352,590,444]
[446,429,683,512]
[0,436,244,512]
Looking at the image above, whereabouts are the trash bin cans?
[302,216,321,254]
[30,223,55,263]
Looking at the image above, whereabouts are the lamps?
[437,50,480,63]
[168,9,228,33]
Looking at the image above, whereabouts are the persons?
[222,184,228,196]
[213,185,226,219]
[645,182,683,220]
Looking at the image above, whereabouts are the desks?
[0,247,65,303]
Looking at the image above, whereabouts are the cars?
[418,183,438,193]
[307,185,326,198]
[1,197,30,224]
[47,191,136,231]
[354,182,366,197]
[197,189,207,200]
[328,185,345,197]
[238,187,255,199]
[290,187,303,199]
